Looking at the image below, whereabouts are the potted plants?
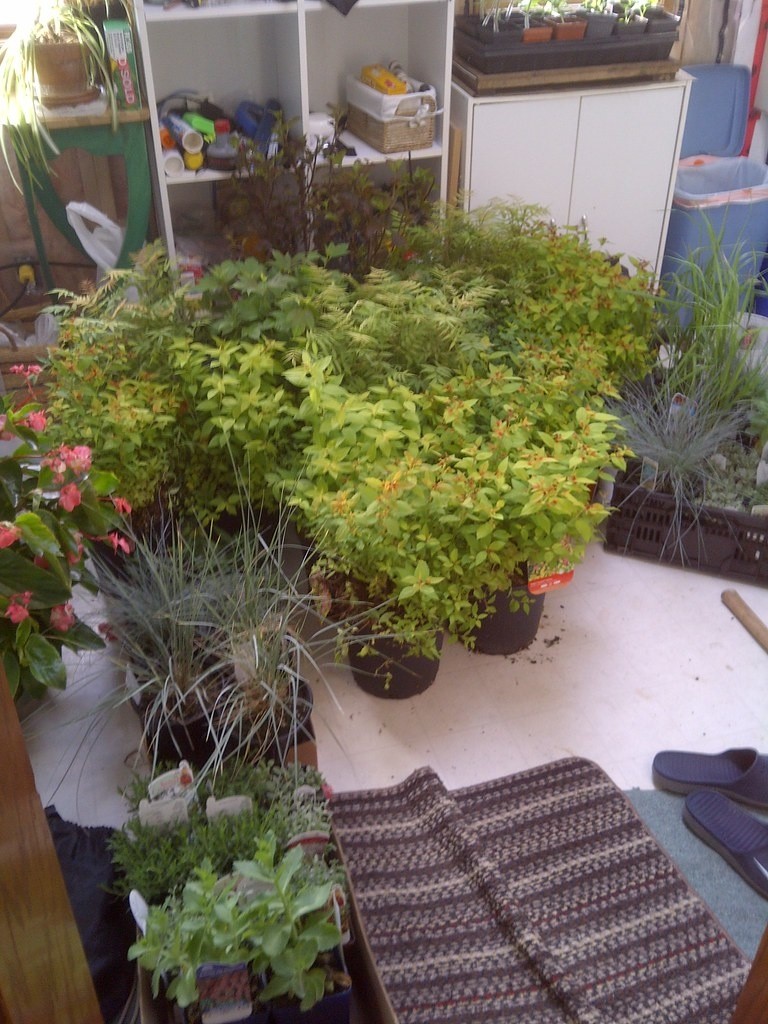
[42,103,768,1024]
[458,0,685,49]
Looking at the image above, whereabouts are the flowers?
[0,361,141,691]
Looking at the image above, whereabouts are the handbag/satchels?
[65,199,146,305]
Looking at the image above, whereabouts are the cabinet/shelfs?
[134,0,453,296]
[451,68,696,298]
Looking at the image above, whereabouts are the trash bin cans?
[650,62,768,338]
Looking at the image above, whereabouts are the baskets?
[346,70,437,154]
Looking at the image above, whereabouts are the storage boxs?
[136,801,399,1024]
[601,457,768,589]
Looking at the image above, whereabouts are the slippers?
[681,789,768,901]
[651,747,768,809]
